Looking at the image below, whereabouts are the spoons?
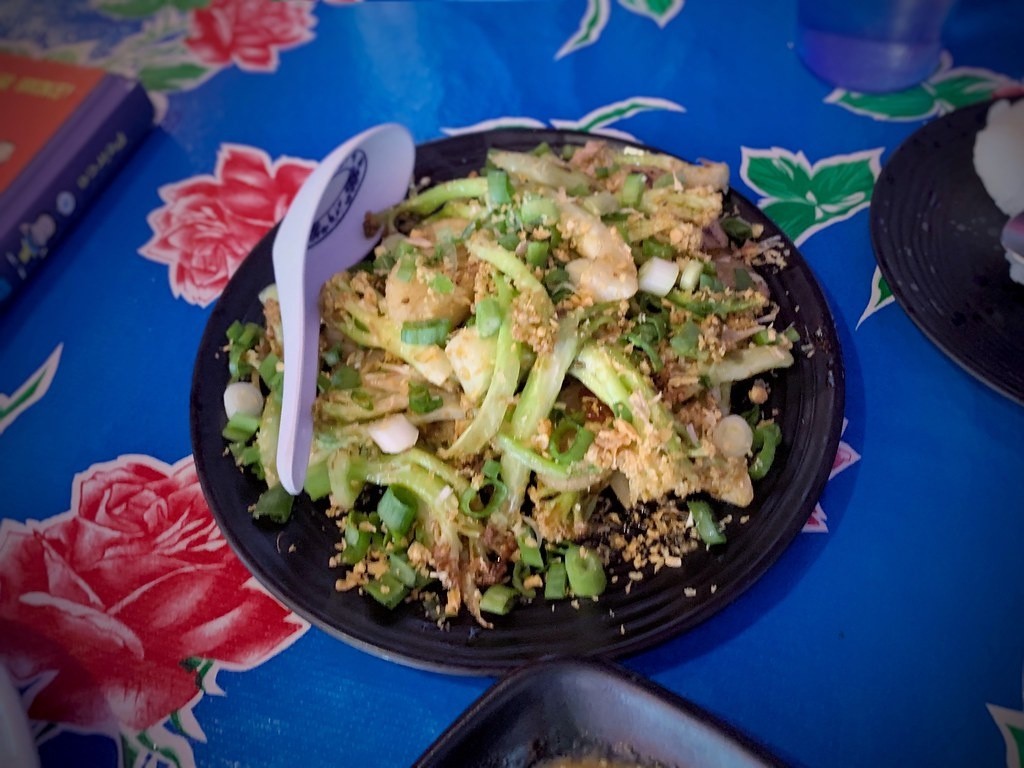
[272,123,415,495]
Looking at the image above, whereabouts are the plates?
[870,93,1024,407]
[191,127,845,677]
[409,655,782,768]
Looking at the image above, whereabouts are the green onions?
[221,140,783,616]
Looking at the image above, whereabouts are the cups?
[797,0,950,92]
